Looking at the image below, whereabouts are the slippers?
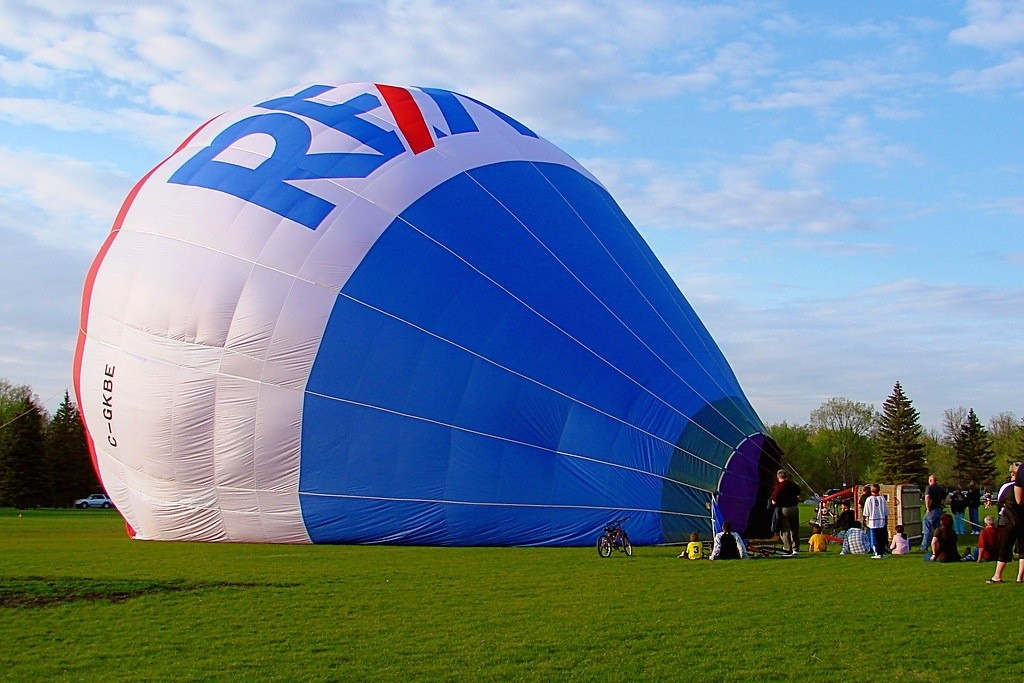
[986,578,1002,584]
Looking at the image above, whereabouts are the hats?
[839,500,850,504]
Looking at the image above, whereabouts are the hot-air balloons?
[71,80,923,548]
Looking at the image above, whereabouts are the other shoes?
[782,549,798,557]
[871,554,885,559]
[956,534,964,536]
[916,549,928,554]
[970,530,980,535]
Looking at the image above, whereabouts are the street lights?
[841,481,847,490]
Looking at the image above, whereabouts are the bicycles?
[596,516,633,558]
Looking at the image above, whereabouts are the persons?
[985,462,1024,583]
[858,484,889,558]
[924,514,962,562]
[949,488,967,535]
[807,525,829,552]
[824,500,854,539]
[1013,463,1024,505]
[889,525,910,554]
[709,522,749,561]
[686,532,705,560]
[965,485,981,534]
[974,515,1001,562]
[983,491,992,508]
[917,474,947,551]
[839,521,872,555]
[771,469,801,554]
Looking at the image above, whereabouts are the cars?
[74,493,112,509]
